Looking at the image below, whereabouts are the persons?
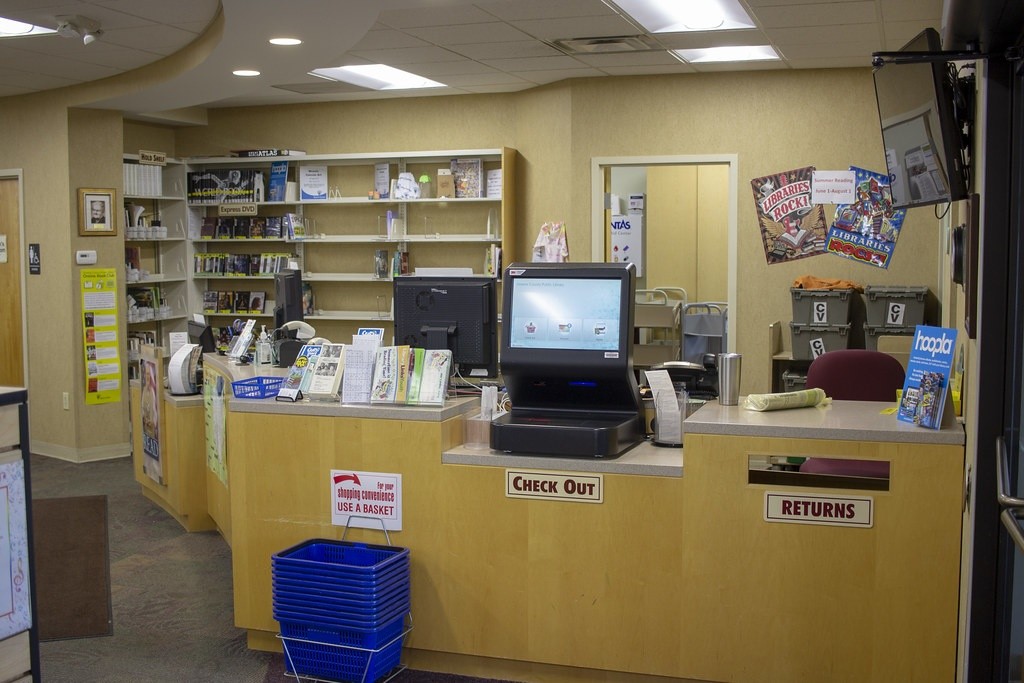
[236,295,248,309]
[211,292,217,303]
[315,362,335,377]
[251,296,261,309]
[323,344,342,358]
[219,295,231,310]
[91,201,105,223]
[140,362,157,439]
[302,284,313,314]
[204,292,211,301]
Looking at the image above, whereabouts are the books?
[201,212,308,240]
[202,291,266,314]
[450,157,485,198]
[375,162,391,198]
[269,161,289,201]
[195,251,294,274]
[299,166,329,199]
[187,167,266,204]
[301,281,315,316]
[486,242,501,278]
[375,249,410,279]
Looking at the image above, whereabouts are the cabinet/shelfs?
[183,145,517,323]
[123,152,187,324]
[634,287,687,370]
[682,302,729,363]
[768,320,915,394]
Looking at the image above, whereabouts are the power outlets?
[63,392,70,410]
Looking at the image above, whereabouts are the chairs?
[805,349,906,402]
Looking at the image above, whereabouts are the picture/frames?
[962,193,980,339]
[77,188,117,236]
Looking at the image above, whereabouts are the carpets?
[30,494,114,642]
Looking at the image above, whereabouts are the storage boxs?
[653,388,687,444]
[789,286,856,325]
[782,365,809,391]
[862,322,915,351]
[863,284,929,327]
[467,410,507,443]
[789,320,852,360]
[231,376,288,399]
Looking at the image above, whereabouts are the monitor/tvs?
[872,27,969,211]
[510,275,621,349]
[188,320,216,368]
[273,268,304,341]
[391,276,499,396]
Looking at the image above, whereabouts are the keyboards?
[449,380,505,391]
